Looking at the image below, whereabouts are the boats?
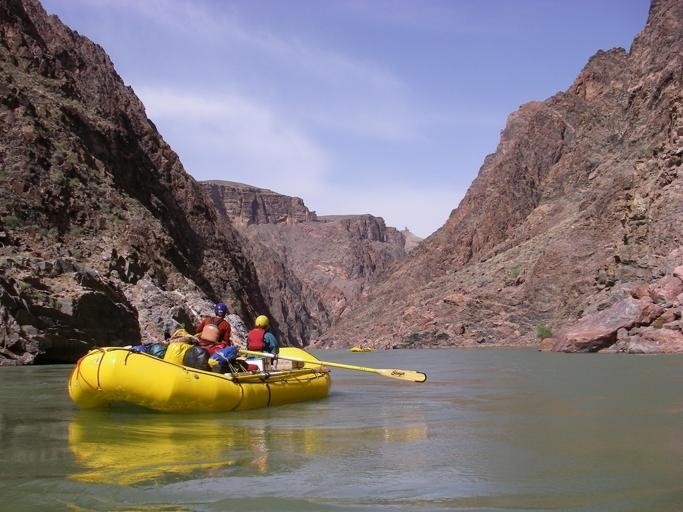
[351,346,373,352]
[68,345,332,415]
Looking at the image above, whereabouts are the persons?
[194,302,244,357]
[245,314,278,366]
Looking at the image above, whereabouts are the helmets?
[215,303,227,314]
[255,315,269,327]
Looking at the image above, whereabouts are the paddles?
[237,350,425,382]
[236,366,331,380]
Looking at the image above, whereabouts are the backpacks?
[200,317,224,343]
